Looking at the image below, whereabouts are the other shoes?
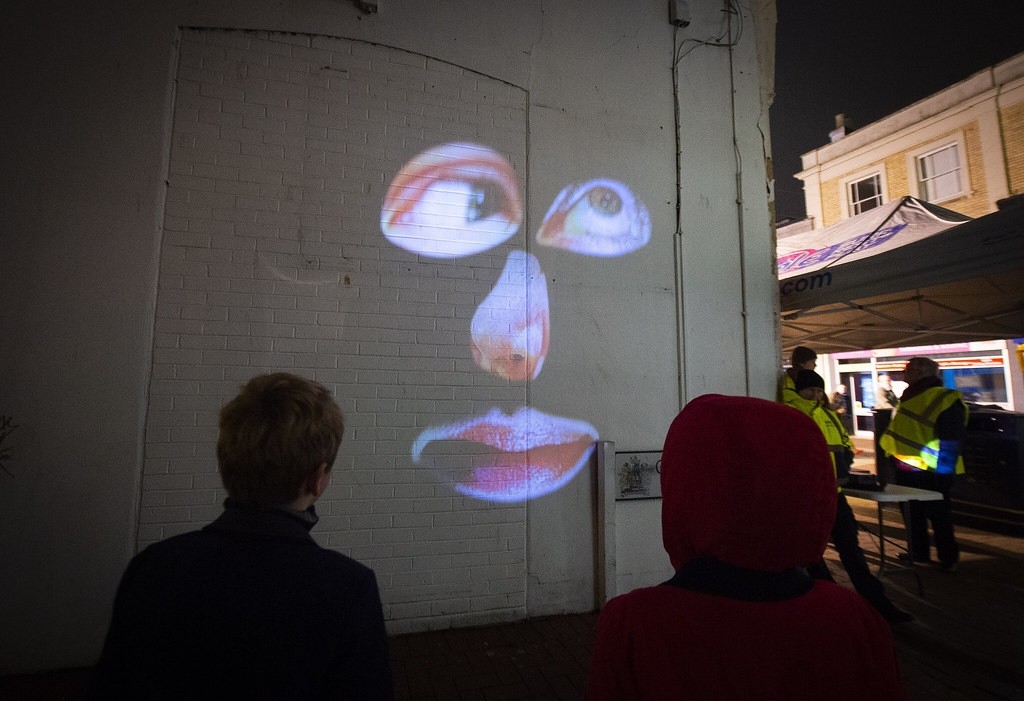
[943,559,957,574]
[895,552,930,562]
[881,605,913,622]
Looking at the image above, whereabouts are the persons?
[93,372,395,701]
[586,393,905,701]
[879,357,970,575]
[777,346,915,624]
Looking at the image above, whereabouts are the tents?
[777,195,1024,355]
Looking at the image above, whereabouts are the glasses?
[655,461,661,473]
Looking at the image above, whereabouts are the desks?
[842,484,944,598]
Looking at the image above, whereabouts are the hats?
[796,369,825,390]
